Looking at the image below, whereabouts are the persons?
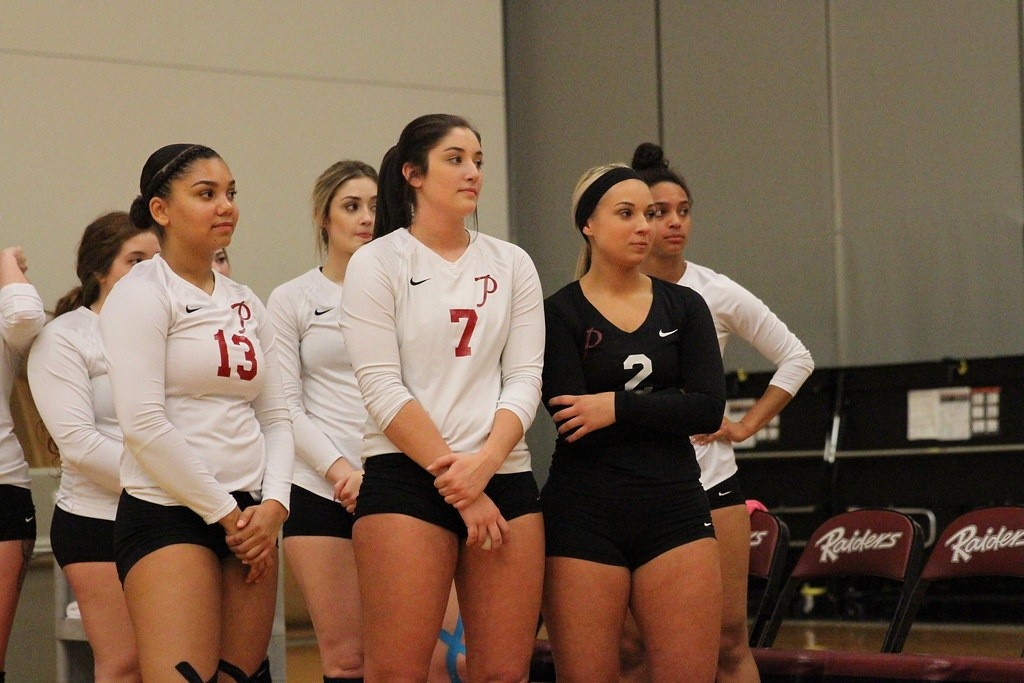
[0,115,547,683]
[539,143,815,683]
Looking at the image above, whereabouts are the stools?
[824,509,1024,682]
[747,508,924,681]
[746,508,789,647]
